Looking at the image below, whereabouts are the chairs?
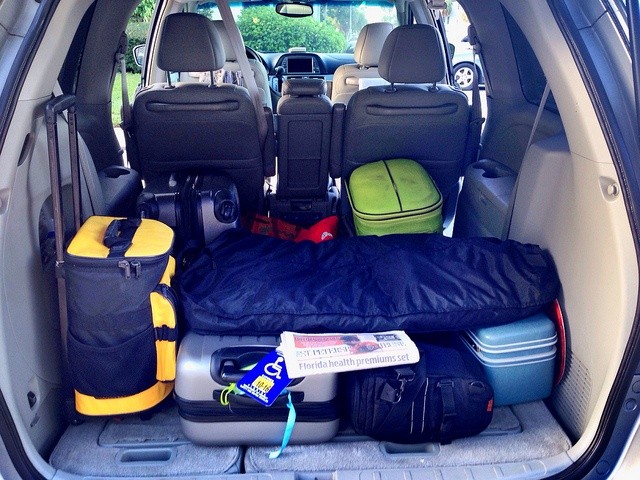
[331,22,395,106]
[180,21,273,111]
[132,12,264,190]
[344,24,469,232]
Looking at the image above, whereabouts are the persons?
[340,336,381,355]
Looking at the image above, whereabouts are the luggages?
[47,94,176,426]
[173,330,341,447]
[137,170,242,245]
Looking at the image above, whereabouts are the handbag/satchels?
[351,342,494,444]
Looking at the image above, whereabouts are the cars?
[447,32,485,90]
